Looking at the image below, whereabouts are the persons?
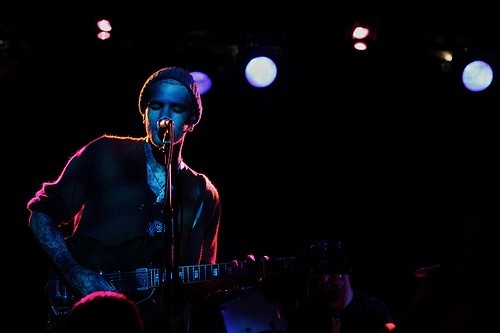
[282,238,397,333]
[26,66,269,332]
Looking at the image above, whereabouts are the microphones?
[156,118,173,131]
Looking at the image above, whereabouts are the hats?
[138,67,203,131]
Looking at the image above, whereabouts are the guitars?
[0,236,351,333]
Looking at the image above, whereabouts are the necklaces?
[147,157,166,192]
